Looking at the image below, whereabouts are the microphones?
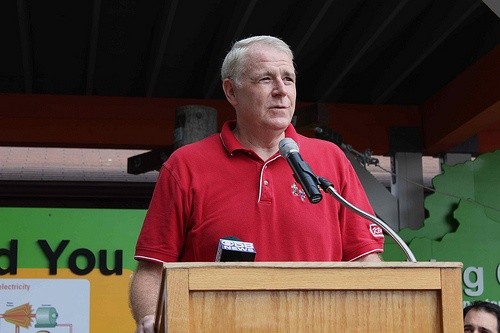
[279,137,323,203]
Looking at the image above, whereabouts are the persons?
[129,35,386,333]
[463,300,500,333]
